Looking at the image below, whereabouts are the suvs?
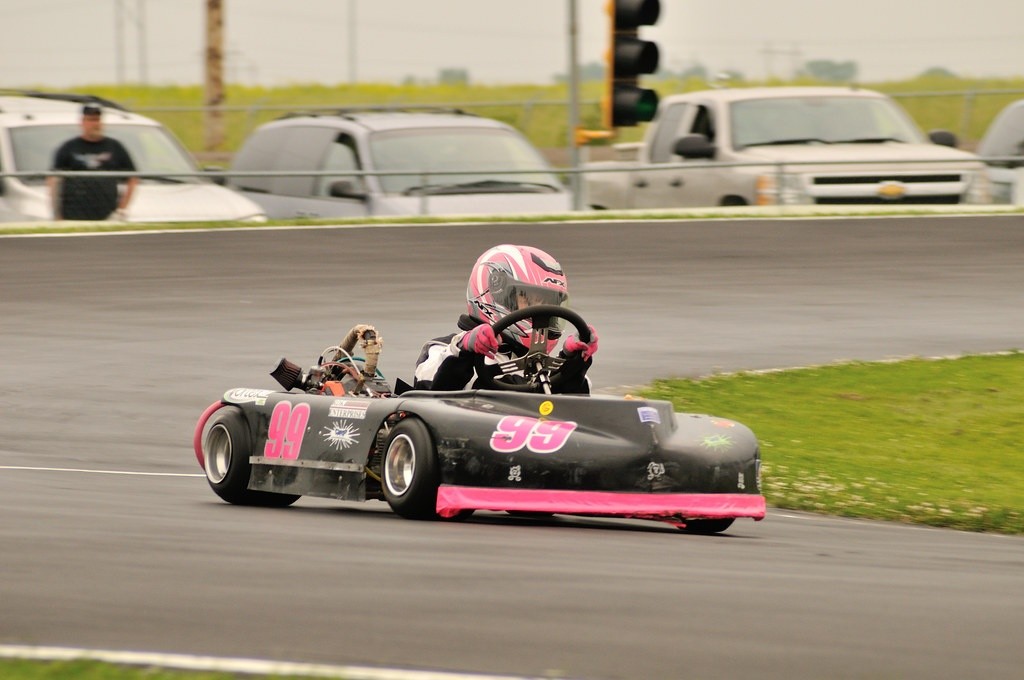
[0,93,268,224]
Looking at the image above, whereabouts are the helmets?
[466,244,569,359]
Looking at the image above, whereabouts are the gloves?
[563,324,599,362]
[464,324,499,360]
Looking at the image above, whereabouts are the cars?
[961,100,1024,205]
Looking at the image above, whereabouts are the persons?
[48,106,139,222]
[414,244,599,397]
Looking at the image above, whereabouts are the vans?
[224,113,610,221]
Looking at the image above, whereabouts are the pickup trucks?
[581,86,986,211]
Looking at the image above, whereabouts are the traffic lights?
[611,0,660,127]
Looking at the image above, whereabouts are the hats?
[84,104,101,116]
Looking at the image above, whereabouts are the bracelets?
[116,208,125,214]
